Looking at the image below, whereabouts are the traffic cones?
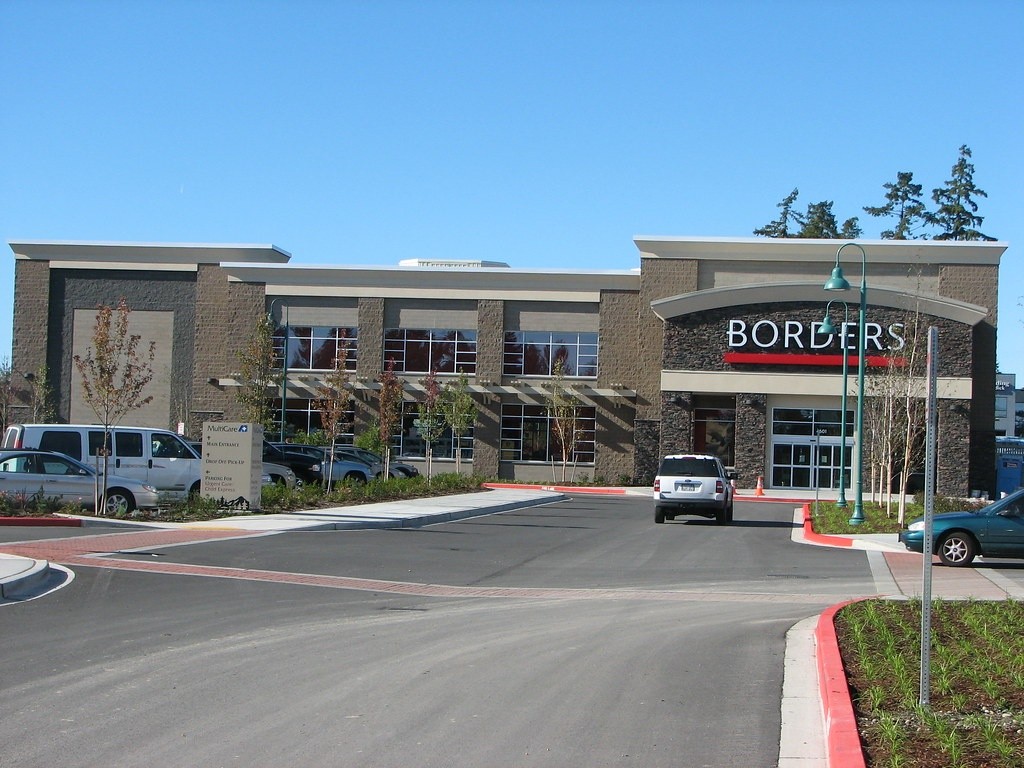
[729,479,739,496]
[754,476,767,496]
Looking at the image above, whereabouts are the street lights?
[266,298,288,445]
[824,242,867,525]
[815,296,849,507]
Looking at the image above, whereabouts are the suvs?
[653,453,738,526]
[152,441,297,493]
[723,465,738,478]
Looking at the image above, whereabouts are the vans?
[1,422,203,506]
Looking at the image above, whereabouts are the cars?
[0,446,161,518]
[898,487,1024,569]
[261,441,420,490]
